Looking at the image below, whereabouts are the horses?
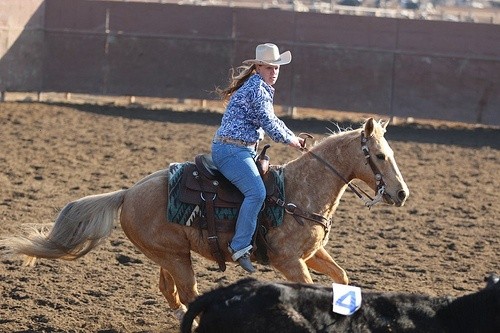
[1,117,409,330]
[181,274,500,333]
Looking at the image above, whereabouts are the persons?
[212,43,307,273]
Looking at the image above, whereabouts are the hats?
[241,43,292,66]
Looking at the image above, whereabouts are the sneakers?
[228,243,255,273]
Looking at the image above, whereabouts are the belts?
[215,134,256,147]
